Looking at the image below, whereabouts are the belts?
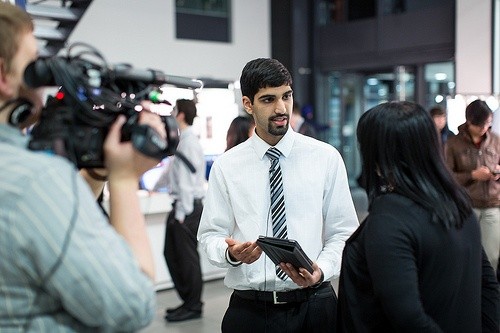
[233,283,333,304]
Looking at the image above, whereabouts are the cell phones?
[492,172,500,175]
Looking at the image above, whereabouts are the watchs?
[310,268,324,288]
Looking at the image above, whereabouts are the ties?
[266,146,292,281]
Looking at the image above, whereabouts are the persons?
[339,100,500,333]
[197,59,360,332]
[225,114,256,154]
[151,96,207,321]
[1,0,170,333]
[428,107,455,150]
[445,98,500,280]
[290,103,335,143]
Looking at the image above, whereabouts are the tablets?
[257,235,314,274]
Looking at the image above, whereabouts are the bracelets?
[225,246,243,267]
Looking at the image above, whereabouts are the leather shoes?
[165,307,202,321]
[167,304,184,314]
